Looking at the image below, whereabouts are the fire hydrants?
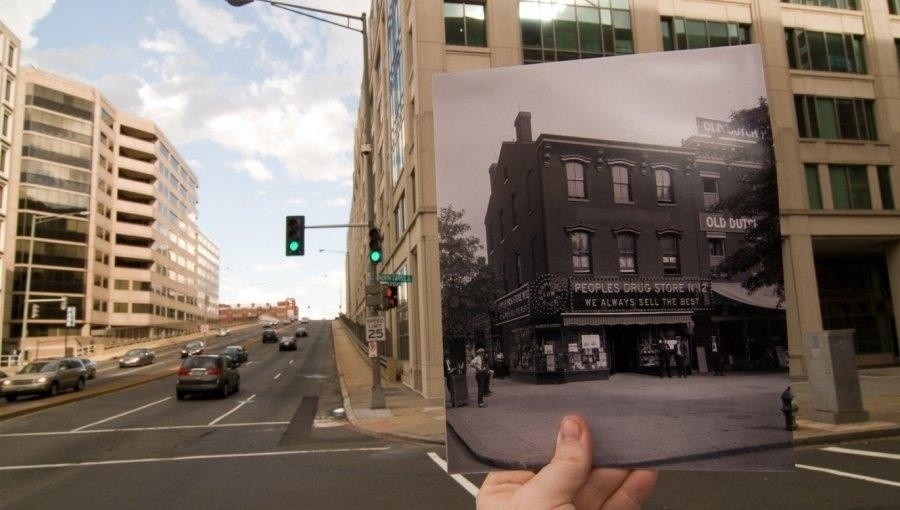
[791,389,799,430]
[781,387,791,431]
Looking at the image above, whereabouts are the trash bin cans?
[444,367,469,408]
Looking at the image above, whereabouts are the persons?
[657,337,674,378]
[674,335,688,378]
[707,335,726,377]
[469,346,489,407]
[474,412,658,510]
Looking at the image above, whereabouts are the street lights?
[225,0,385,413]
[20,209,90,358]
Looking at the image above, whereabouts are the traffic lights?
[30,303,40,320]
[386,285,398,308]
[369,229,384,265]
[286,216,304,256]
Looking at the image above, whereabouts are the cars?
[175,341,248,401]
[263,328,308,351]
[119,348,156,367]
[0,356,96,402]
[301,316,311,324]
[217,327,231,338]
[261,316,296,327]
